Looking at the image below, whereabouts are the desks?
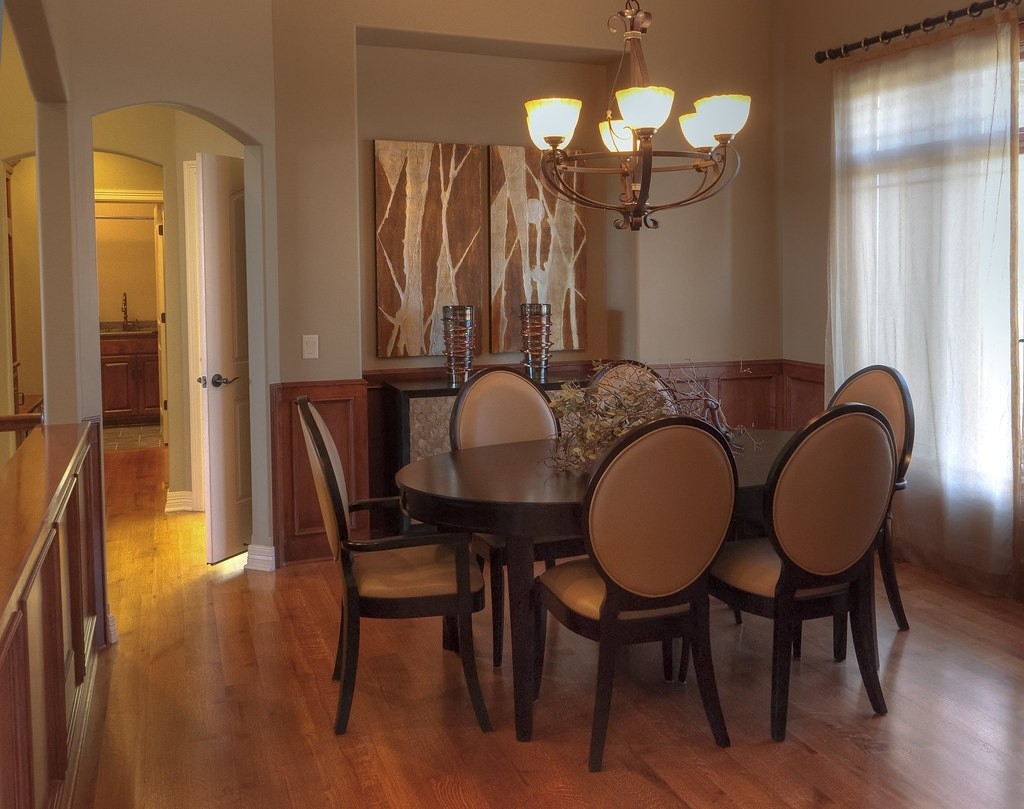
[395,428,806,742]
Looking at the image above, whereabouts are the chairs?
[733,366,916,663]
[679,402,897,739]
[593,361,680,429]
[530,416,731,776]
[295,393,494,737]
[450,364,586,668]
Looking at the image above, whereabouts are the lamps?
[524,0,750,230]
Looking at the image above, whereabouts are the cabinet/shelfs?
[382,377,589,536]
[100,337,160,421]
